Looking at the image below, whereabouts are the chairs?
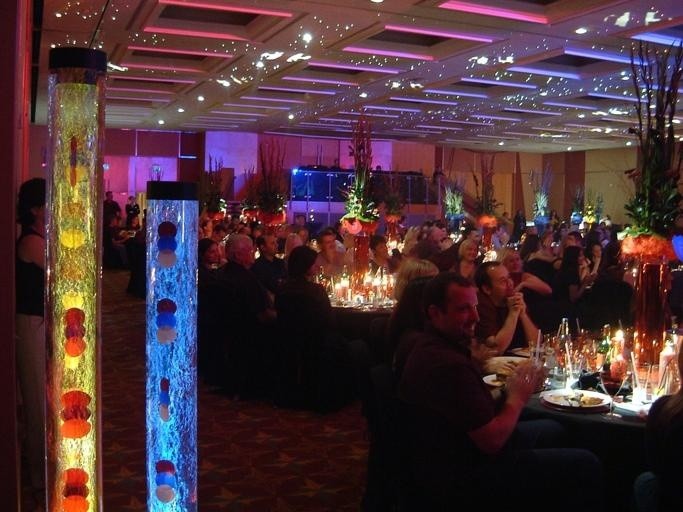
[123,237,683,512]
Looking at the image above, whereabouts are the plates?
[482,374,507,387]
[543,389,611,408]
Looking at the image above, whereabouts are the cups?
[528,340,545,369]
[635,363,669,409]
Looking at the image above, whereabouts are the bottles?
[319,264,393,305]
[544,317,678,389]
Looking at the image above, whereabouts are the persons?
[14,158,682,512]
